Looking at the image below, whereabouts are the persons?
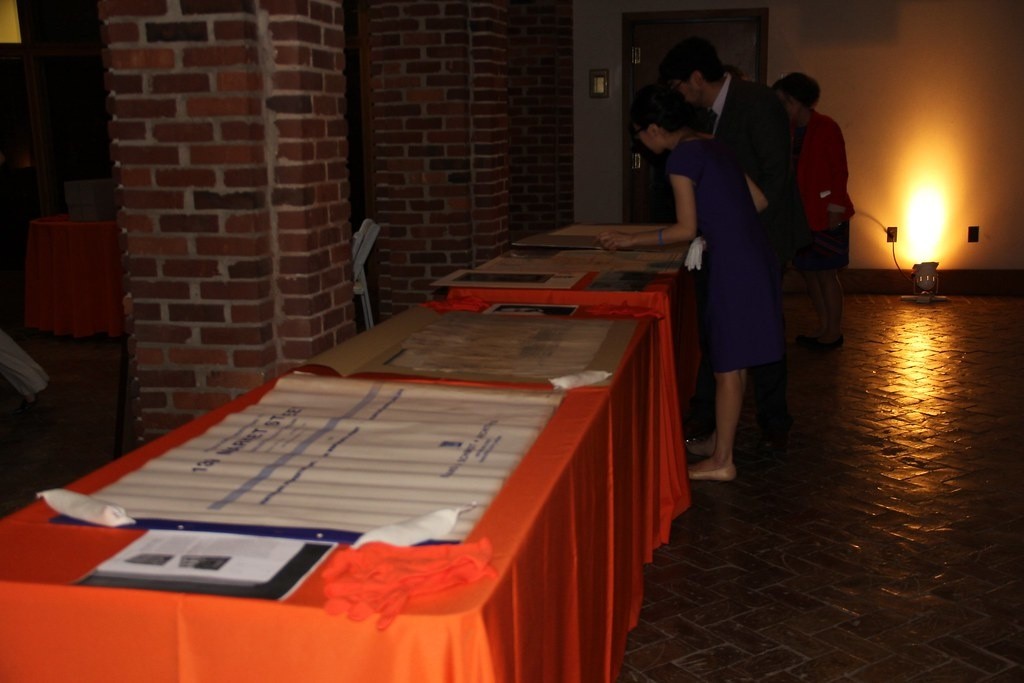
[773,72,854,350]
[656,36,791,452]
[593,85,785,482]
[0,330,50,415]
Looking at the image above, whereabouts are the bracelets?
[659,229,663,246]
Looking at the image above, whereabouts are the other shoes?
[795,334,843,352]
[687,458,736,481]
[756,434,789,453]
[683,438,714,457]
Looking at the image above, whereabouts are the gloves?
[684,236,705,271]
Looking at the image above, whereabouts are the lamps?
[900,262,946,300]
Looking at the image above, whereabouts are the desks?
[0,220,706,683]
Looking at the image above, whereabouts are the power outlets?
[968,226,979,243]
[887,227,897,242]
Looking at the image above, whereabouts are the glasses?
[630,125,649,141]
[669,77,688,96]
